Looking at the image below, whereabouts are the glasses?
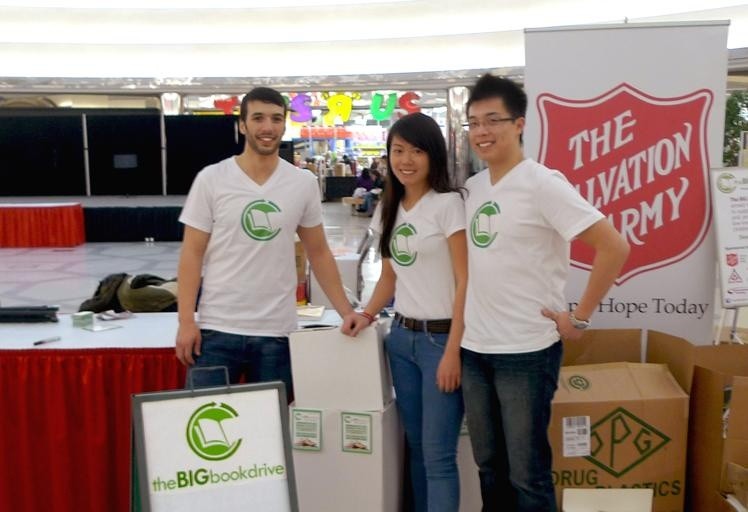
[461,115,518,131]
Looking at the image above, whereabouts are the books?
[297,304,327,318]
[298,320,339,332]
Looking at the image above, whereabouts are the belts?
[392,310,452,336]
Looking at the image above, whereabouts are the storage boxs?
[289,385,406,512]
[548,329,747,511]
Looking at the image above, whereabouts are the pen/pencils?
[33,337,61,345]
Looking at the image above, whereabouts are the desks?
[0,203,86,248]
[0,309,392,512]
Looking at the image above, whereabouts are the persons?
[460,71,633,511]
[171,86,381,405]
[339,112,469,511]
[293,150,387,217]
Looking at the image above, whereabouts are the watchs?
[567,308,592,331]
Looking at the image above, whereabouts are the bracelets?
[358,311,375,325]
